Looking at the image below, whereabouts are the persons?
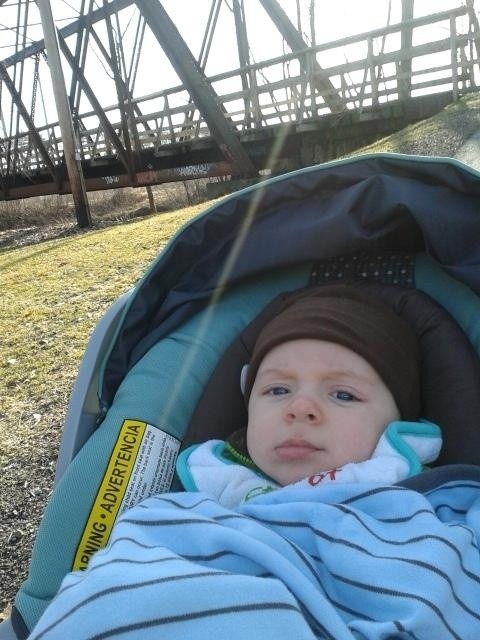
[239,286,425,489]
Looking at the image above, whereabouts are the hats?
[244,287,422,422]
